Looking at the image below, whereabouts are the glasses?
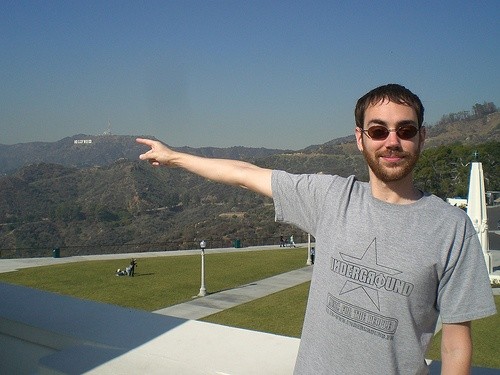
[359,123,422,140]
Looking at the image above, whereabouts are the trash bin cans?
[52,246,60,258]
[235,239,240,248]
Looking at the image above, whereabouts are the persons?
[310,247,315,265]
[280,233,286,247]
[290,235,295,248]
[284,239,289,247]
[136,84,499,375]
[128,257,137,277]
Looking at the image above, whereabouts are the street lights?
[198,240,209,297]
